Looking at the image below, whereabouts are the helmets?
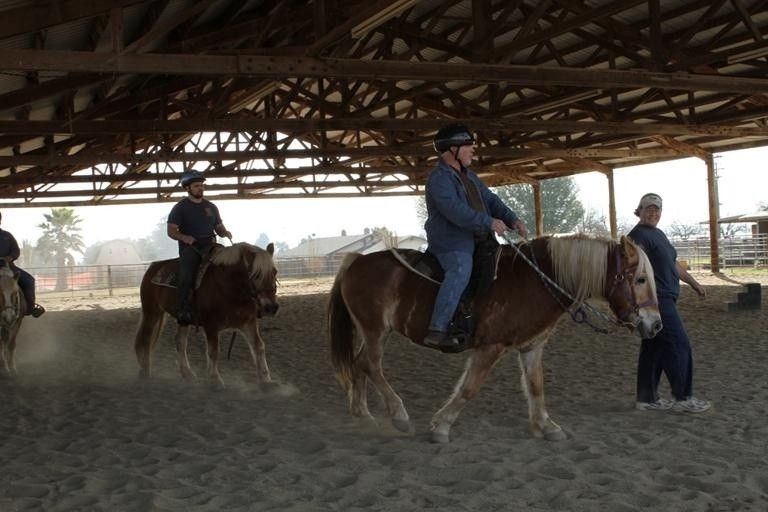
[432,121,476,153]
[180,169,206,188]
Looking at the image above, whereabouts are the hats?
[640,193,662,208]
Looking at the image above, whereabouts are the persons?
[0,212,45,318]
[166,169,232,325]
[422,124,527,353]
[627,194,712,414]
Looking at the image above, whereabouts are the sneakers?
[423,329,462,354]
[175,309,192,326]
[635,397,674,411]
[672,395,712,414]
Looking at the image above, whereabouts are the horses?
[0,253,29,380]
[326,231,667,447]
[131,241,282,390]
